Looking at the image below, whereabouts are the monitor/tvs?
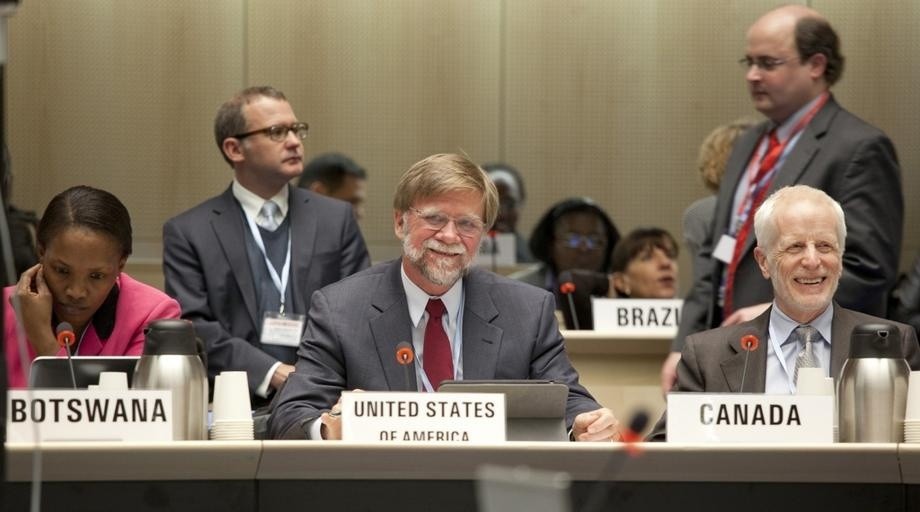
[29,354,139,388]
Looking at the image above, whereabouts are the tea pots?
[835,323,912,442]
[129,319,208,439]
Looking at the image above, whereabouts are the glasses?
[738,55,800,70]
[558,230,607,251]
[407,206,481,237]
[234,122,308,142]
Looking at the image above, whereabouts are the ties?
[760,130,780,162]
[795,326,819,387]
[262,202,278,231]
[422,299,454,392]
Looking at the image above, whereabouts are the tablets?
[435,378,569,442]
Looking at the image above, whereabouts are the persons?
[163,86,372,409]
[2,143,44,283]
[480,161,539,261]
[609,226,680,297]
[660,5,903,401]
[681,119,756,281]
[643,186,918,443]
[265,148,620,440]
[3,184,184,388]
[527,198,621,328]
[297,152,367,221]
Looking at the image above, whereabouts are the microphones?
[736,326,760,393]
[395,340,415,391]
[56,322,78,389]
[558,270,582,330]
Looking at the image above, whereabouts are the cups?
[903,371,920,443]
[823,378,839,441]
[215,371,254,440]
[796,367,824,395]
[210,375,219,440]
